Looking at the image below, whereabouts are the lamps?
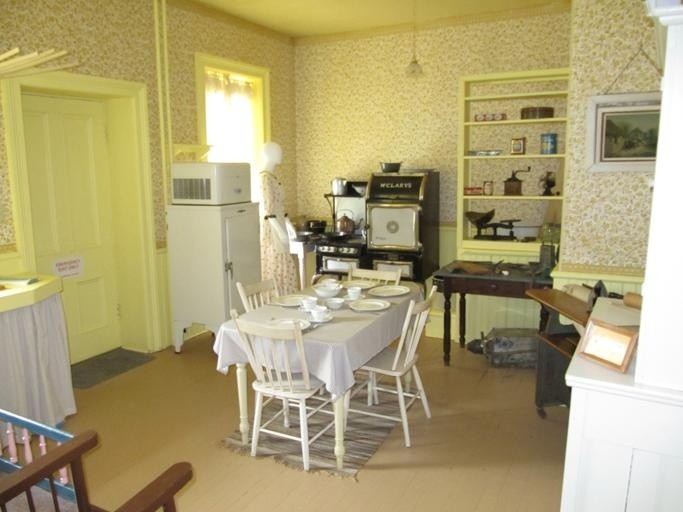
[405,53,425,81]
[536,222,556,268]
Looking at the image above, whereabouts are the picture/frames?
[577,316,639,375]
[584,90,663,174]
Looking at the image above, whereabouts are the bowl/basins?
[327,298,344,309]
[322,278,336,284]
[312,283,343,297]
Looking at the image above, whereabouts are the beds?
[0,408,99,512]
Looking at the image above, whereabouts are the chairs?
[235,273,325,396]
[343,284,437,448]
[348,262,402,405]
[229,308,335,471]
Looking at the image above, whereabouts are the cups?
[311,306,332,321]
[348,287,362,300]
[298,297,317,312]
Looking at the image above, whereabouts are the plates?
[307,315,333,323]
[343,294,365,301]
[299,305,319,313]
[273,295,310,307]
[268,318,311,331]
[368,285,410,297]
[349,299,390,312]
[343,281,376,289]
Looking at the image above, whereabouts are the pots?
[321,231,347,241]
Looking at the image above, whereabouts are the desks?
[524,288,594,417]
[432,260,556,367]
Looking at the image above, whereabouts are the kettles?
[335,209,363,237]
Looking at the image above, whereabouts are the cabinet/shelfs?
[456,66,570,344]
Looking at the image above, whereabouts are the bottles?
[483,180,493,196]
[540,133,557,154]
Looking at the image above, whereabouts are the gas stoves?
[317,235,368,258]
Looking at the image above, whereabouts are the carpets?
[220,377,417,484]
